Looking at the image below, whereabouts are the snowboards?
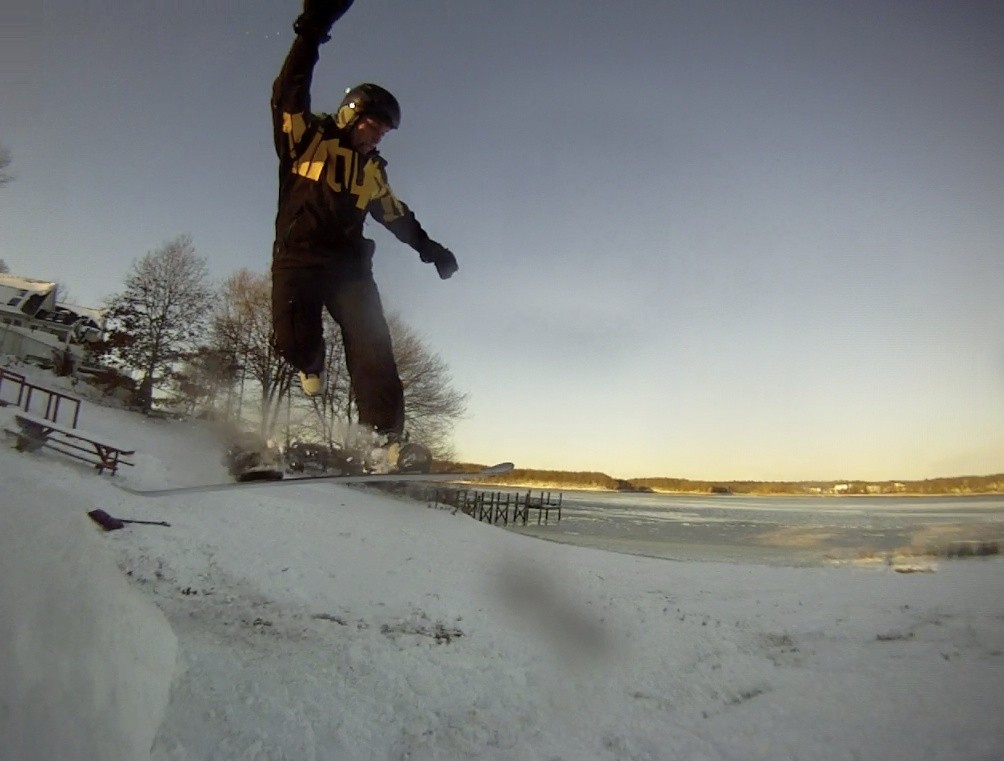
[105,458,516,497]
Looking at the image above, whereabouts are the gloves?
[293,0,354,44]
[420,243,458,280]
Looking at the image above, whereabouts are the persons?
[268,0,459,472]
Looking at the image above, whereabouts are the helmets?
[338,83,400,130]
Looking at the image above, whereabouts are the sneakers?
[372,438,426,473]
[300,366,327,397]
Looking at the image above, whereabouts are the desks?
[15,412,136,475]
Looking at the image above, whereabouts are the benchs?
[4,426,135,476]
